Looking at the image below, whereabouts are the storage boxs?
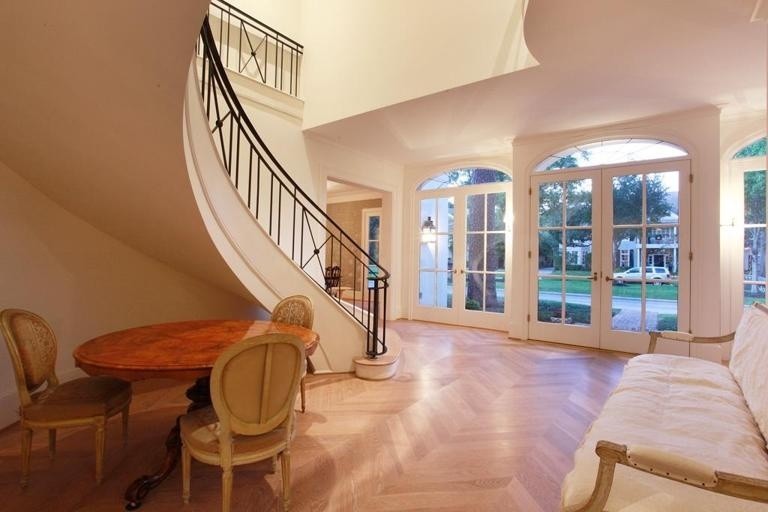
[561,299,768,512]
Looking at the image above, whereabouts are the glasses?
[421,216,435,244]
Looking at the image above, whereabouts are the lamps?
[0,309,133,489]
[271,295,314,412]
[325,266,341,296]
[175,332,306,512]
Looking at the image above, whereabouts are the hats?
[613,266,673,286]
